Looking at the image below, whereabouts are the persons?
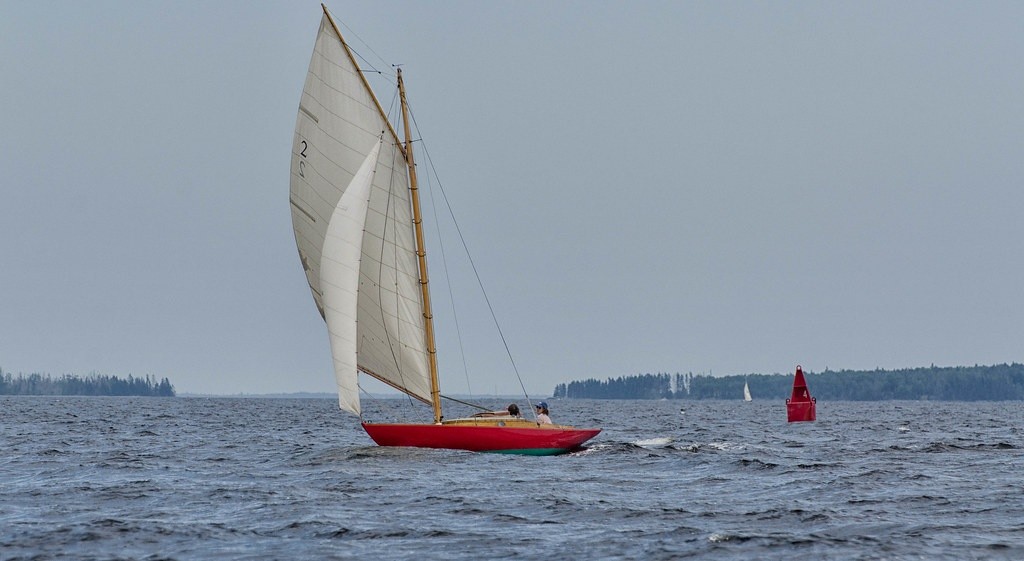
[534,401,553,425]
[508,403,523,418]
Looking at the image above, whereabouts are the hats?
[534,401,547,409]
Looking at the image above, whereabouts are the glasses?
[536,406,541,409]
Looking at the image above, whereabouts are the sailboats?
[289,3,604,457]
